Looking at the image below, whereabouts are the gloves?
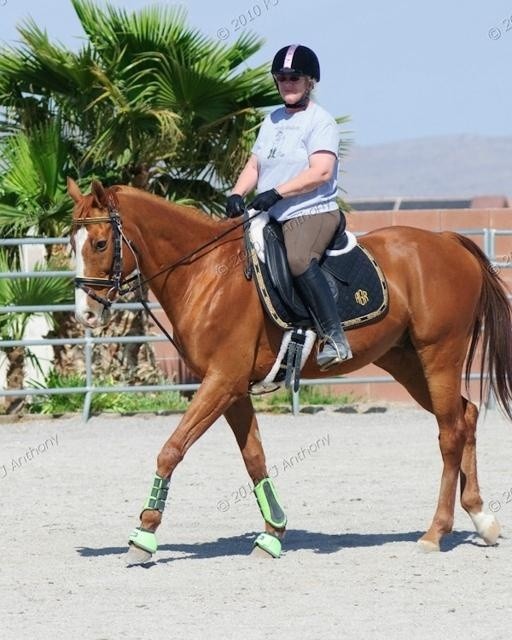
[226,194,245,218]
[247,188,283,211]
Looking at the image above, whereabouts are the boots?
[289,257,348,366]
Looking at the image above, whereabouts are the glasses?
[278,76,299,82]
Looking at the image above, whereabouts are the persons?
[226,45,353,368]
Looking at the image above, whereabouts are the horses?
[63,171,512,567]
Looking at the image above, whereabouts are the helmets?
[271,44,320,82]
